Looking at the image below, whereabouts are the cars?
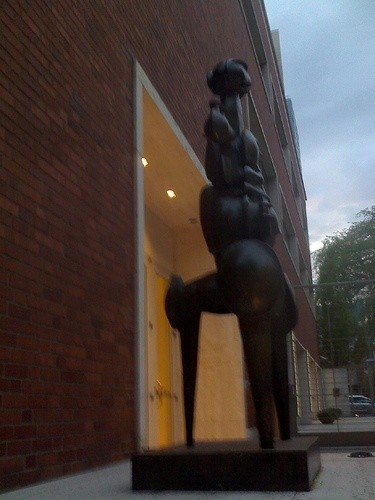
[348,394,373,409]
